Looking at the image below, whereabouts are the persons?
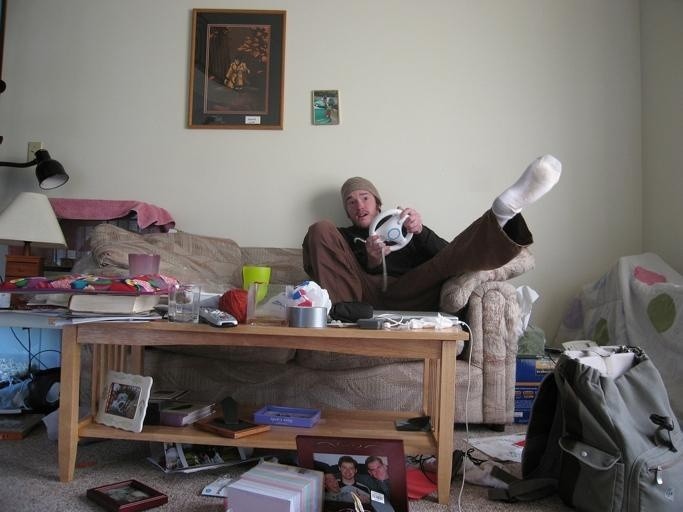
[335,455,371,503]
[219,55,250,90]
[321,470,341,500]
[364,456,390,499]
[300,152,564,321]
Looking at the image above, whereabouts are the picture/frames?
[196,394,272,441]
[185,9,288,131]
[310,89,340,127]
[85,479,169,511]
[92,369,154,433]
[295,428,409,512]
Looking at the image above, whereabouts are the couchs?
[86,226,520,435]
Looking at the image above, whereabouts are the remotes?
[198,307,238,327]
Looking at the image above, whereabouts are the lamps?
[1,192,68,282]
[3,147,70,191]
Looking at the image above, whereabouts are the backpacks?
[521,339,682,511]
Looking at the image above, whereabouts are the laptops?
[373,309,458,326]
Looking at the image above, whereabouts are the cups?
[166,282,201,328]
[127,252,160,276]
[246,283,294,328]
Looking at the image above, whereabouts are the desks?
[0,308,78,332]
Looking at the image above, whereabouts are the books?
[64,289,161,315]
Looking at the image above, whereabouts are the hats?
[341,176,382,203]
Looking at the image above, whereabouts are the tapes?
[288,306,327,327]
